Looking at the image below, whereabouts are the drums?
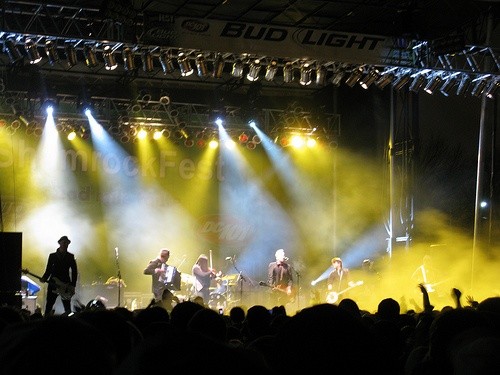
[206,293,228,313]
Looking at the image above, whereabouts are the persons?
[328,258,355,289]
[0,284,500,375]
[41,236,77,315]
[268,249,293,306]
[144,250,170,300]
[410,255,431,278]
[192,254,217,303]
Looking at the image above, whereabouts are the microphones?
[225,257,232,260]
[114,247,119,258]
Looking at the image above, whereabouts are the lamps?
[0,31,500,152]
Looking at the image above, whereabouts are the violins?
[209,268,217,278]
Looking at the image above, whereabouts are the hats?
[58,236,71,244]
[331,257,342,264]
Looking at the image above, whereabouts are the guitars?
[417,279,446,294]
[259,280,303,301]
[21,268,76,300]
[326,280,364,304]
